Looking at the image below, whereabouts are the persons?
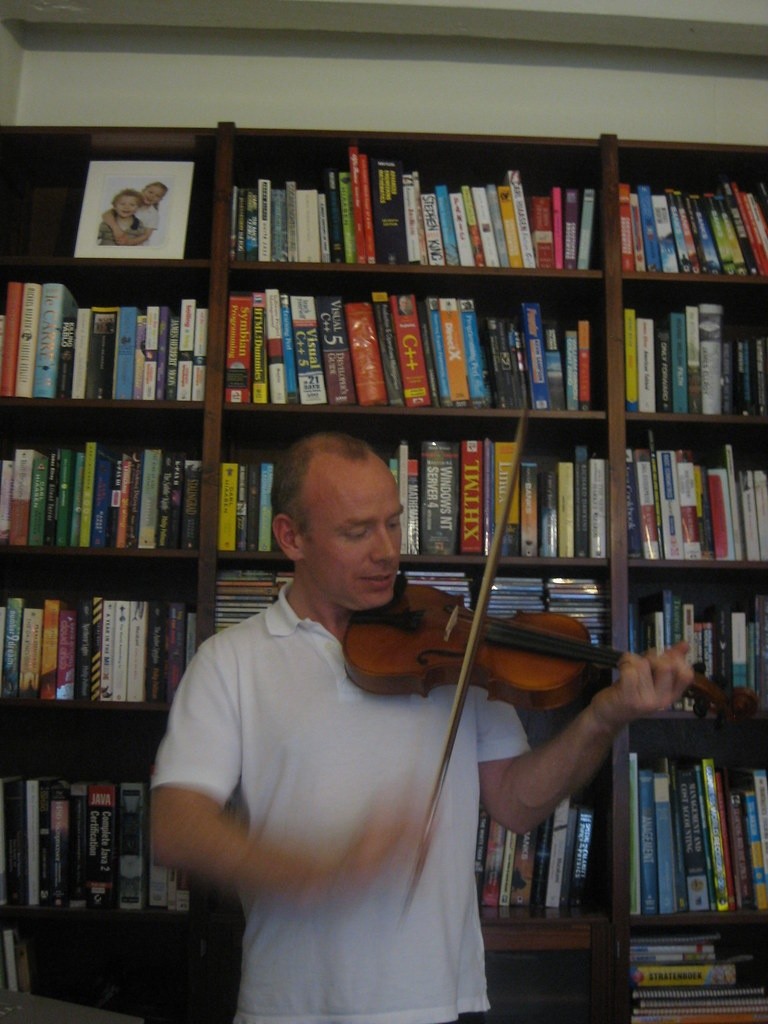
[98,189,146,245]
[152,428,697,1024]
[102,181,169,246]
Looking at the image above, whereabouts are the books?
[387,442,768,561]
[4,774,195,911]
[630,929,768,1024]
[0,572,293,702]
[226,141,768,275]
[477,746,768,910]
[3,927,30,994]
[401,569,768,715]
[1,277,768,417]
[0,440,275,555]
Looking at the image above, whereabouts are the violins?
[341,574,760,732]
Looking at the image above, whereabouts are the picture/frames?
[73,159,194,260]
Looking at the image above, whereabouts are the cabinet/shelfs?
[0,122,768,1023]
